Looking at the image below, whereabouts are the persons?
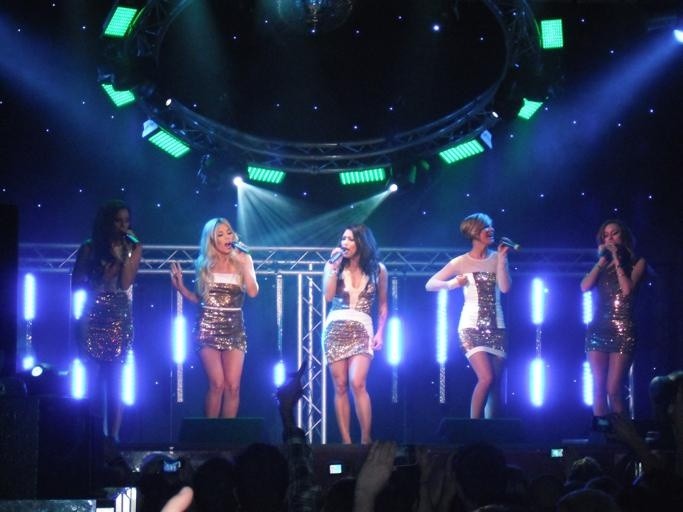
[422,210,515,424]
[319,222,390,449]
[576,216,648,417]
[167,216,260,420]
[67,198,143,447]
[3,412,398,511]
[415,414,682,511]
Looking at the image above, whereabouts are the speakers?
[181,417,265,451]
[434,418,520,447]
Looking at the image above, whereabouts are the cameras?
[162,460,182,473]
[328,463,343,475]
[592,416,610,432]
[391,446,420,466]
[550,448,564,458]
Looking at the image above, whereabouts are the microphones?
[122,230,139,245]
[495,235,520,250]
[231,240,252,254]
[599,243,613,256]
[329,247,345,264]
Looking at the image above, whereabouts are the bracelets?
[327,268,338,275]
[595,261,602,271]
[614,263,623,271]
[122,251,132,259]
[446,277,461,291]
